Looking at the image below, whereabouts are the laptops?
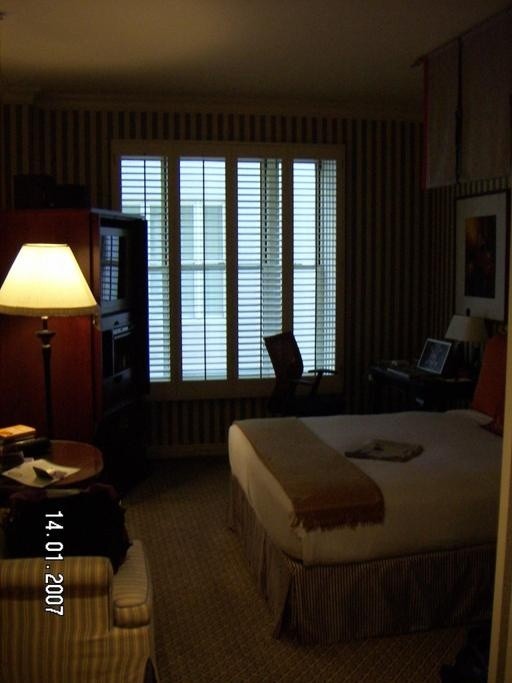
[387,338,452,379]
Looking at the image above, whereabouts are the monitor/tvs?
[99,227,134,315]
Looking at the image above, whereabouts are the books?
[344,438,425,463]
[0,423,81,489]
[386,364,430,380]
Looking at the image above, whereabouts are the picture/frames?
[453,187,511,326]
[415,337,454,376]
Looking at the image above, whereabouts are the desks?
[367,362,471,413]
[1,438,105,491]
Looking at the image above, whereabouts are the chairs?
[265,330,347,416]
[0,540,158,682]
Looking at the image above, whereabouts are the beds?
[225,410,503,646]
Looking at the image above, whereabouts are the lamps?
[1,243,102,435]
[443,313,490,380]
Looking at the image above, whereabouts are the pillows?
[472,334,506,437]
[3,484,132,574]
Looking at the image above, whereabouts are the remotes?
[3,437,49,452]
